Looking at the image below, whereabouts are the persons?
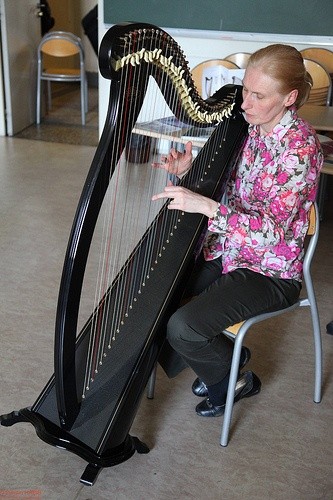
[147,43,324,417]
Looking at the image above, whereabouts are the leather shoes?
[192,346,250,397]
[195,370,261,416]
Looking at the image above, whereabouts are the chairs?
[36,31,89,126]
[145,195,323,447]
[190,48,333,138]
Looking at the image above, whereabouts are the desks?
[132,116,333,224]
